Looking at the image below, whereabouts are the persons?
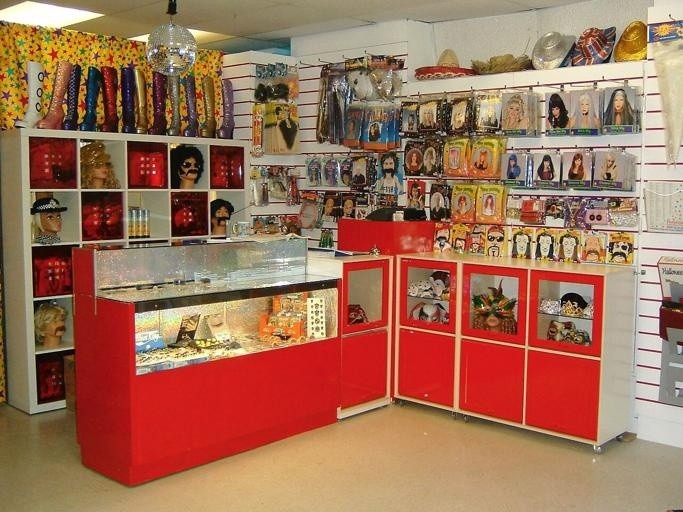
[32,302,67,347]
[277,105,295,150]
[321,90,641,227]
[79,142,123,188]
[471,277,516,334]
[28,196,70,243]
[168,146,205,190]
[208,198,234,236]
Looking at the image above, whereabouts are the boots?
[37,62,81,130]
[149,72,166,135]
[167,75,181,136]
[218,79,234,138]
[100,66,118,132]
[80,67,101,130]
[200,76,216,137]
[183,76,198,137]
[15,61,43,127]
[121,68,147,134]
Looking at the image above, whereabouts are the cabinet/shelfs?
[394,249,637,445]
[303,254,394,420]
[0,128,251,415]
[71,246,343,488]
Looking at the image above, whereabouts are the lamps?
[147,0,197,77]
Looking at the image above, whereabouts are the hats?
[414,49,527,79]
[31,199,69,212]
[528,25,614,70]
[617,23,647,60]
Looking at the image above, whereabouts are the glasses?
[182,162,201,168]
[94,161,112,169]
